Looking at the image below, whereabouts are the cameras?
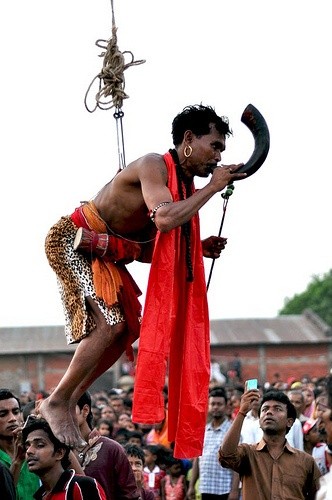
[23,416,35,429]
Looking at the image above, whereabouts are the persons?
[39,104,269,459]
[0,350,332,500]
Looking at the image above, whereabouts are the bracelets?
[237,411,248,417]
[151,201,171,224]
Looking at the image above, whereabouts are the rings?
[227,167,233,172]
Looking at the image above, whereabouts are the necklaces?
[167,149,194,283]
[75,450,87,459]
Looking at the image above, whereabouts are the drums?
[73,227,95,252]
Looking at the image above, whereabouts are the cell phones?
[247,379,257,391]
